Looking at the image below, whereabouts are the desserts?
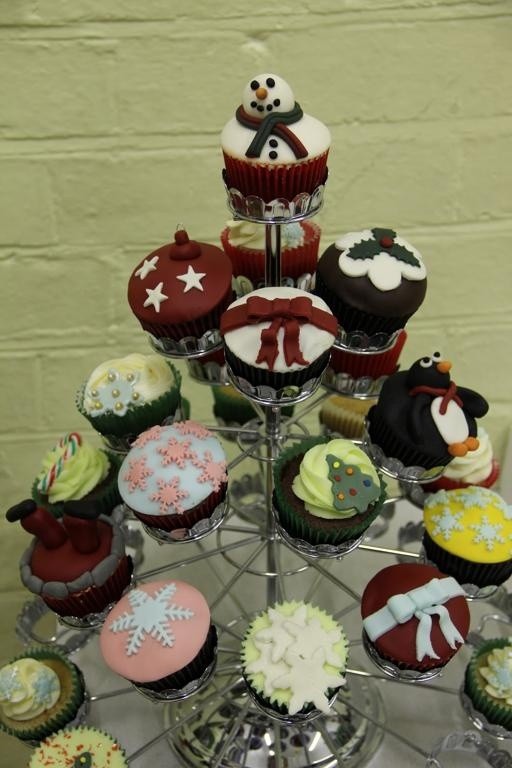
[0,73,512,768]
